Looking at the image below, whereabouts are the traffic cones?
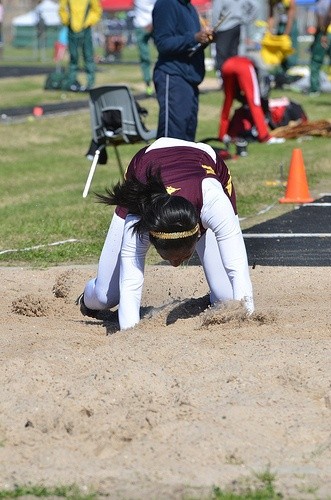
[278,148,315,203]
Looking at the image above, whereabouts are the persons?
[132,1,156,95]
[206,0,331,92]
[76,137,254,329]
[218,57,286,144]
[153,0,217,141]
[58,0,103,90]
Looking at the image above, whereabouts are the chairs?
[82,85,158,198]
